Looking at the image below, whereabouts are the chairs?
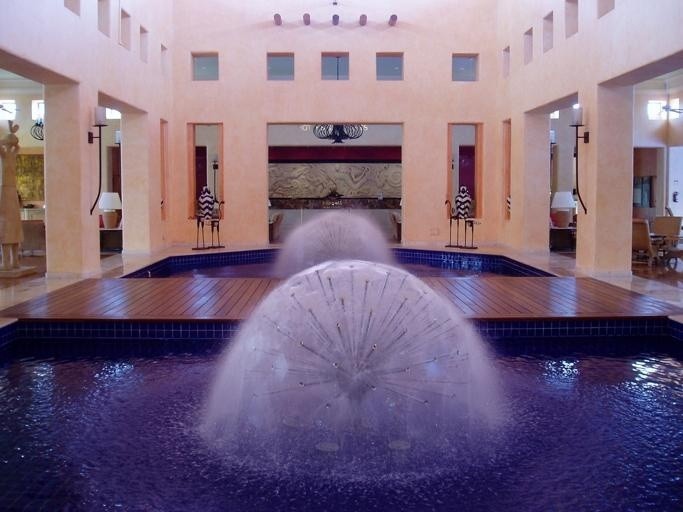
[632,215,683,270]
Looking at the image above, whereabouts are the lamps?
[632,144,658,208]
[210,152,221,200]
[568,105,591,215]
[98,191,123,229]
[86,104,109,216]
[312,57,366,145]
[114,129,122,156]
[551,129,558,164]
[549,190,574,228]
